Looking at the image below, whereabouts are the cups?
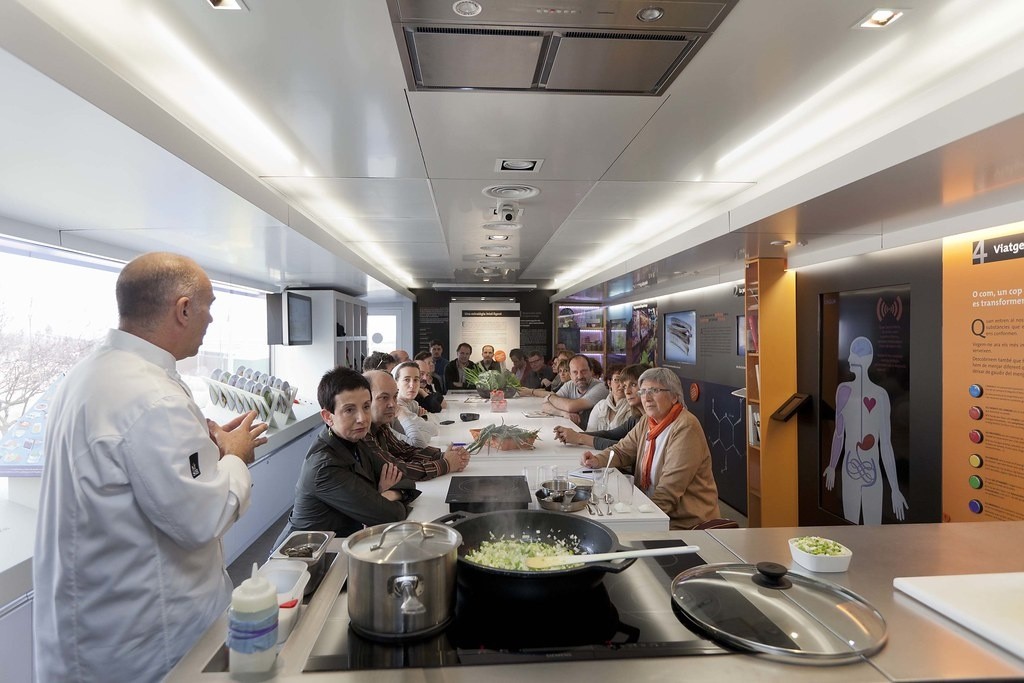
[617,475,634,505]
[594,470,609,498]
[524,466,538,491]
[538,466,552,487]
[553,468,568,481]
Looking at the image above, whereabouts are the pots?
[535,488,591,513]
[431,509,638,594]
[342,520,462,645]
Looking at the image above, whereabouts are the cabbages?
[475,370,503,390]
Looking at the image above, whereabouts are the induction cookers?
[303,539,802,673]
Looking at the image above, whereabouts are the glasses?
[637,388,670,395]
[611,378,620,383]
[375,353,390,369]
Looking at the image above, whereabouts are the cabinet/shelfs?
[557,306,606,376]
[744,256,800,526]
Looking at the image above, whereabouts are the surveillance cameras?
[502,209,514,221]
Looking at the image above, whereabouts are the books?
[522,410,553,418]
[570,465,614,481]
[464,398,489,403]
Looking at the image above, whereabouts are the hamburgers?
[668,317,692,354]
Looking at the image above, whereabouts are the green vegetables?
[506,373,521,389]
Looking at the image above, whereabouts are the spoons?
[603,494,614,515]
[589,492,603,516]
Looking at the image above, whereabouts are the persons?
[268,339,722,554]
[34,252,269,683]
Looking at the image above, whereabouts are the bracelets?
[547,393,553,400]
[532,388,535,397]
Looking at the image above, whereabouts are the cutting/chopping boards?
[893,573,1024,661]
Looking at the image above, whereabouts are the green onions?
[794,535,842,556]
[466,415,542,455]
[461,364,482,386]
[466,531,586,571]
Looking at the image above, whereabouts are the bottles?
[225,562,279,673]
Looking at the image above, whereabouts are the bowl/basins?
[789,538,853,572]
[540,481,576,496]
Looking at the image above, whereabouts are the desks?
[405,389,670,534]
